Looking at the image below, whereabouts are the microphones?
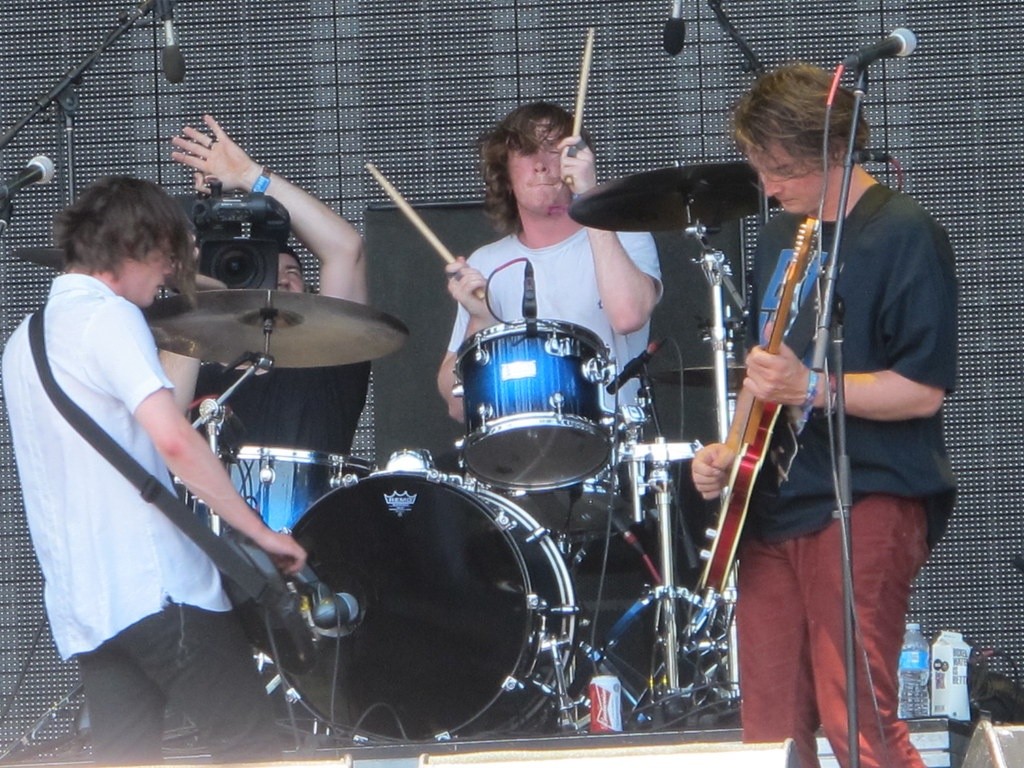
[156,0,186,83]
[663,0,685,54]
[844,28,917,67]
[0,156,55,200]
[220,400,248,440]
[310,593,359,629]
[606,337,665,395]
[521,262,537,317]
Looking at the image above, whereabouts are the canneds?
[589,674,623,735]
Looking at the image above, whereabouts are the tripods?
[570,439,729,727]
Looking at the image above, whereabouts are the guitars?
[701,210,821,598]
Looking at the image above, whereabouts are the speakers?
[959,720,1024,768]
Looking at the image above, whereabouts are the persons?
[437,101,664,662]
[691,65,960,768]
[1,175,307,768]
[155,115,371,507]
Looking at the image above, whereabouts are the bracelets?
[801,369,818,408]
[250,167,272,195]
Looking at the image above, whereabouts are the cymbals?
[649,364,748,390]
[141,286,409,368]
[566,161,782,234]
[15,244,67,273]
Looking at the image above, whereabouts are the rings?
[208,139,218,148]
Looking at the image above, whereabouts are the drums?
[465,412,644,543]
[271,469,578,745]
[207,445,376,550]
[454,315,617,492]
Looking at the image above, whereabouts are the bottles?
[897,623,930,719]
